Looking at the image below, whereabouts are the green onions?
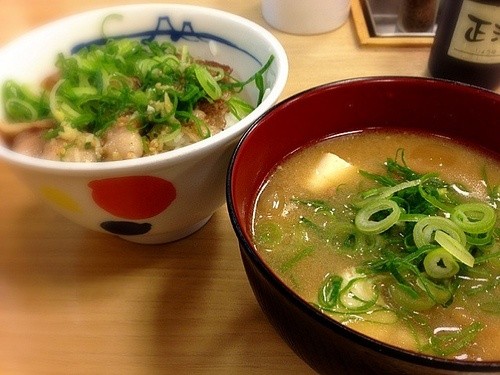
[2,35,274,138]
[258,151,500,353]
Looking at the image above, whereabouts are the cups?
[425,0,499,86]
[262,1,350,35]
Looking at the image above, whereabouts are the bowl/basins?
[227,75,499,373]
[0,2,290,247]
